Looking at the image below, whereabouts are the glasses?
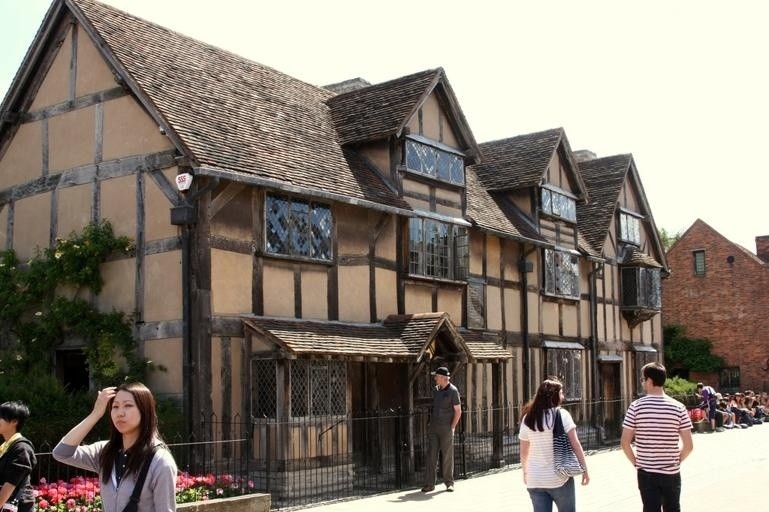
[640,377,647,382]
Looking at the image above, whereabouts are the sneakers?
[709,429,715,432]
[422,485,435,492]
[447,485,453,491]
[716,427,725,432]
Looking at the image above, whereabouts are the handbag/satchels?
[554,433,584,478]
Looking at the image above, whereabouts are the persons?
[52,382,177,512]
[694,382,769,432]
[518,379,590,512]
[0,401,37,512]
[422,367,462,491]
[621,361,693,512]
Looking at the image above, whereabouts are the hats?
[431,367,450,376]
